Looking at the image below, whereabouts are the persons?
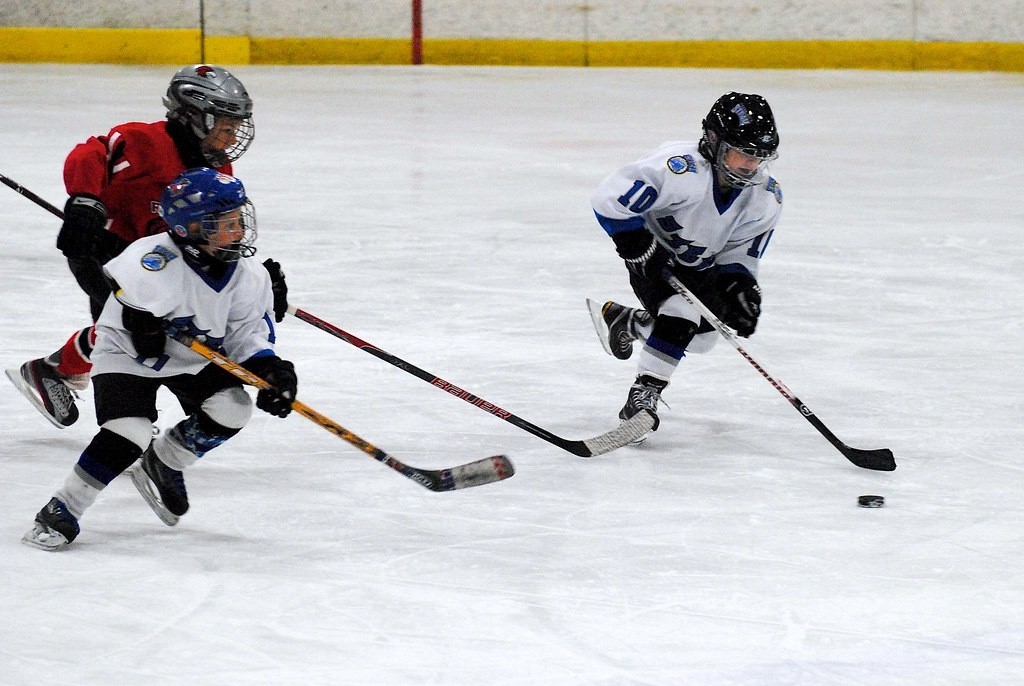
[20,167,297,551]
[5,63,255,477]
[585,92,783,446]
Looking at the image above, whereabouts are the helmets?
[158,167,255,252]
[704,92,779,158]
[163,65,253,146]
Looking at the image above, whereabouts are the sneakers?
[130,437,190,527]
[19,495,82,550]
[619,371,670,446]
[585,297,654,361]
[6,358,81,430]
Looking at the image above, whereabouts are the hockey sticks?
[276,282,659,458]
[0,172,64,219]
[167,327,515,495]
[668,274,895,471]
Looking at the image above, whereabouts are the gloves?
[263,257,290,322]
[125,307,169,359]
[720,270,764,339]
[57,192,109,267]
[252,356,299,417]
[609,227,679,285]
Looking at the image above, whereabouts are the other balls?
[856,492,884,509]
